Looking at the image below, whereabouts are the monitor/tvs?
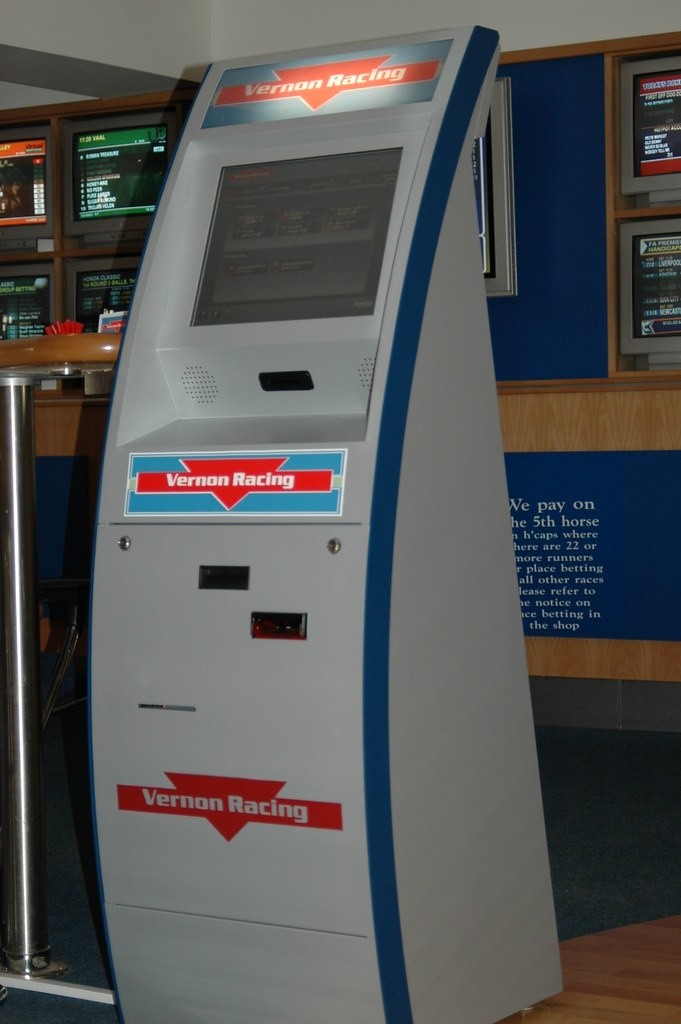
[0,125,52,251]
[0,262,55,338]
[61,111,177,246]
[63,256,143,334]
[619,55,681,209]
[188,147,404,327]
[620,219,681,370]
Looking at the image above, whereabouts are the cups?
[50,362,81,376]
